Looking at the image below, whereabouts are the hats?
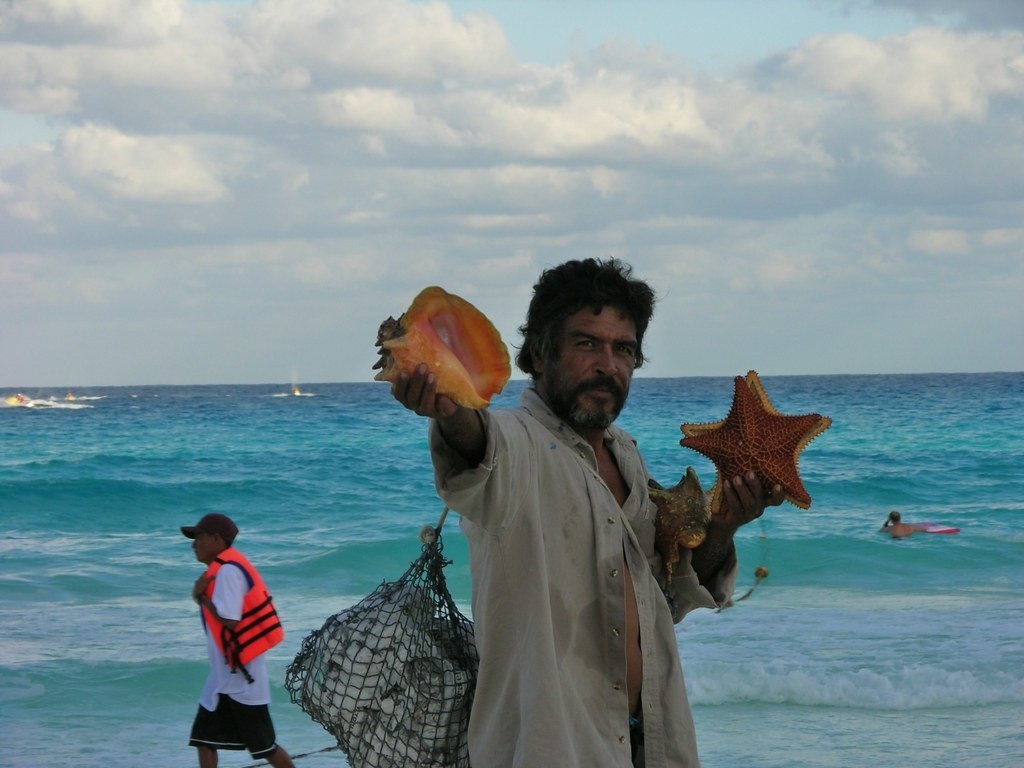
[180,513,239,544]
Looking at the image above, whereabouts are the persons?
[879,511,927,538]
[179,514,296,768]
[388,257,787,768]
